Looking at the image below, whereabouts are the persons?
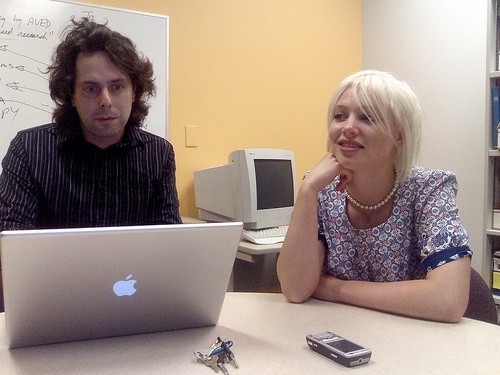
[0,15,182,232]
[276,69,473,323]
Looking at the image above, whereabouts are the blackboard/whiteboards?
[1,0,169,170]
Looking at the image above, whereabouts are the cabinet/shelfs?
[482,0,500,325]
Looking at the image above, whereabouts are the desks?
[0,292,500,375]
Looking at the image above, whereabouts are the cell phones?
[305,331,372,367]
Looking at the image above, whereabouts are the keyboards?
[244,225,290,245]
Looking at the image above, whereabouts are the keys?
[193,335,240,375]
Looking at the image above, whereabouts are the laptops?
[0,222,243,348]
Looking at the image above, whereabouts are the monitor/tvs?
[192,148,298,229]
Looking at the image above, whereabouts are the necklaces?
[343,161,400,210]
